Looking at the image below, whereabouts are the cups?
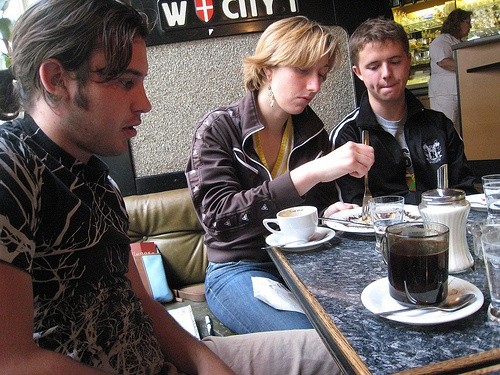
[368,195,405,260]
[381,221,450,307]
[262,206,318,244]
[472,174,500,322]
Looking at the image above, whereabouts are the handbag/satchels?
[130,241,207,302]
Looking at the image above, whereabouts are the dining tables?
[262,194,500,375]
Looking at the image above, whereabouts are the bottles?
[419,163,474,274]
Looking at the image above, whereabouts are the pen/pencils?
[204,313,213,336]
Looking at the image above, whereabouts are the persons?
[184,13,375,333]
[320,12,481,210]
[1,1,373,375]
[426,5,473,141]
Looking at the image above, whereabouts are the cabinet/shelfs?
[390,0,500,161]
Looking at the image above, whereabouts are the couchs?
[123,188,234,339]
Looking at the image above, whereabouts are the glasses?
[464,18,471,25]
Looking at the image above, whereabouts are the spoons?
[362,293,475,321]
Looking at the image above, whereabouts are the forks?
[361,130,377,223]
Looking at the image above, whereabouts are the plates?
[265,226,335,251]
[465,192,488,212]
[360,274,485,326]
[326,204,423,236]
